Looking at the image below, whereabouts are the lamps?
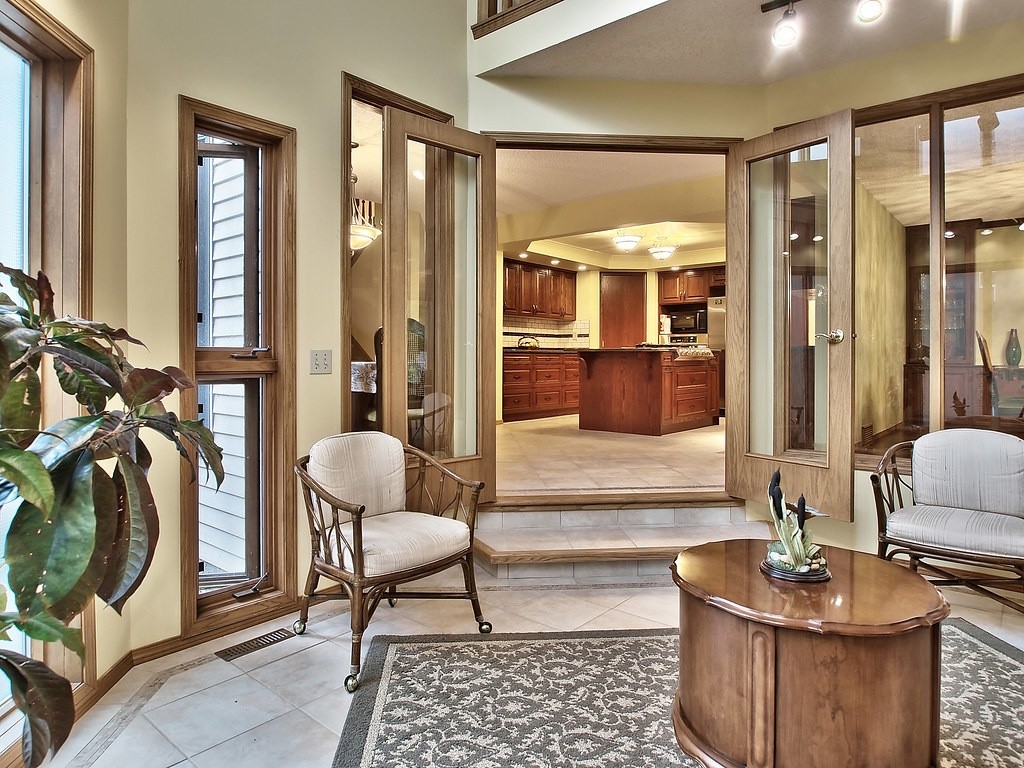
[856,0,884,23]
[350,142,382,258]
[771,2,799,50]
[647,236,680,263]
[609,228,647,255]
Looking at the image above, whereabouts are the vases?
[1005,328,1022,368]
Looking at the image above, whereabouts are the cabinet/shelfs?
[903,364,1024,425]
[658,267,719,306]
[709,265,726,287]
[906,263,975,365]
[519,260,548,318]
[502,354,579,423]
[548,266,578,321]
[503,257,519,316]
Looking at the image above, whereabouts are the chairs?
[361,317,425,443]
[292,430,493,694]
[870,428,1024,614]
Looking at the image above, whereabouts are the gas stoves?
[636,334,708,348]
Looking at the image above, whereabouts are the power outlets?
[573,333,577,340]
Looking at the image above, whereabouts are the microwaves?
[670,310,707,334]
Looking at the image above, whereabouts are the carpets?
[332,616,1024,768]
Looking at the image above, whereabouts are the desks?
[997,399,1024,416]
[670,538,952,768]
[350,361,377,410]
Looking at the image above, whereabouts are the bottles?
[1006,329,1022,365]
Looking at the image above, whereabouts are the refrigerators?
[707,297,726,350]
